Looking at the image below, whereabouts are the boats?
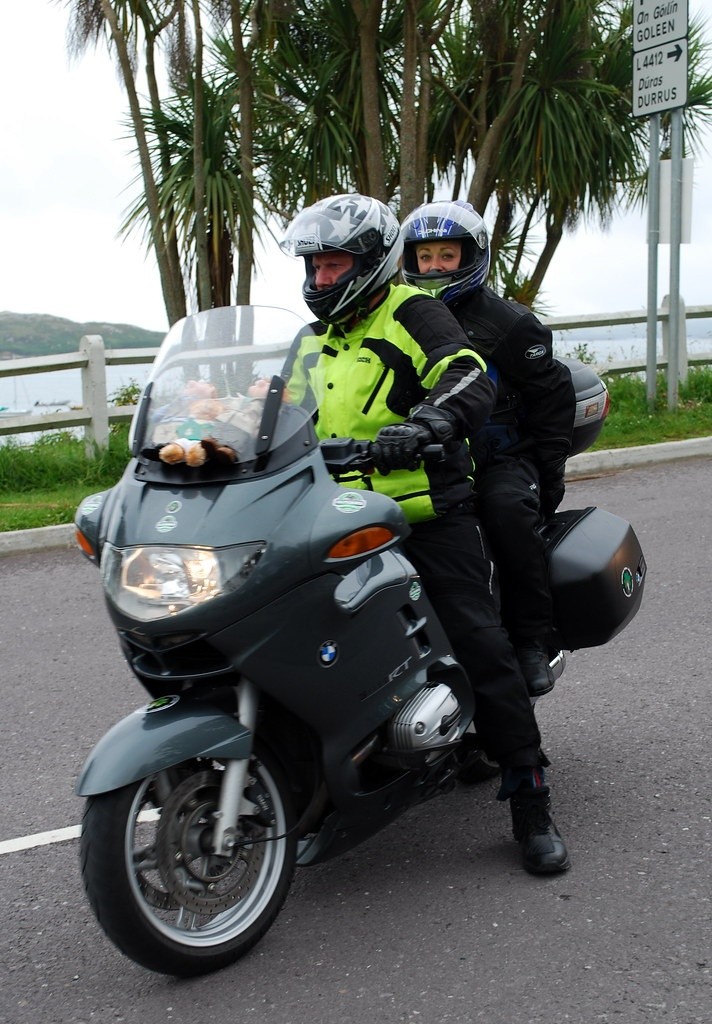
[0,402,30,420]
[32,400,70,406]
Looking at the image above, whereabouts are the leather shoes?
[519,635,554,698]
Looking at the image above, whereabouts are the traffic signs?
[623,0,690,123]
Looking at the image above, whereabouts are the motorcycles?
[62,303,654,981]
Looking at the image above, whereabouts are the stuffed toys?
[160,395,226,466]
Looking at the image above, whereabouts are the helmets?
[399,200,492,307]
[281,193,405,325]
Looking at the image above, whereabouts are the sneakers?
[510,786,571,875]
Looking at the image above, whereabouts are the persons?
[277,194,572,881]
[400,198,581,699]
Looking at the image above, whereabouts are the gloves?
[532,436,571,522]
[375,404,456,476]
